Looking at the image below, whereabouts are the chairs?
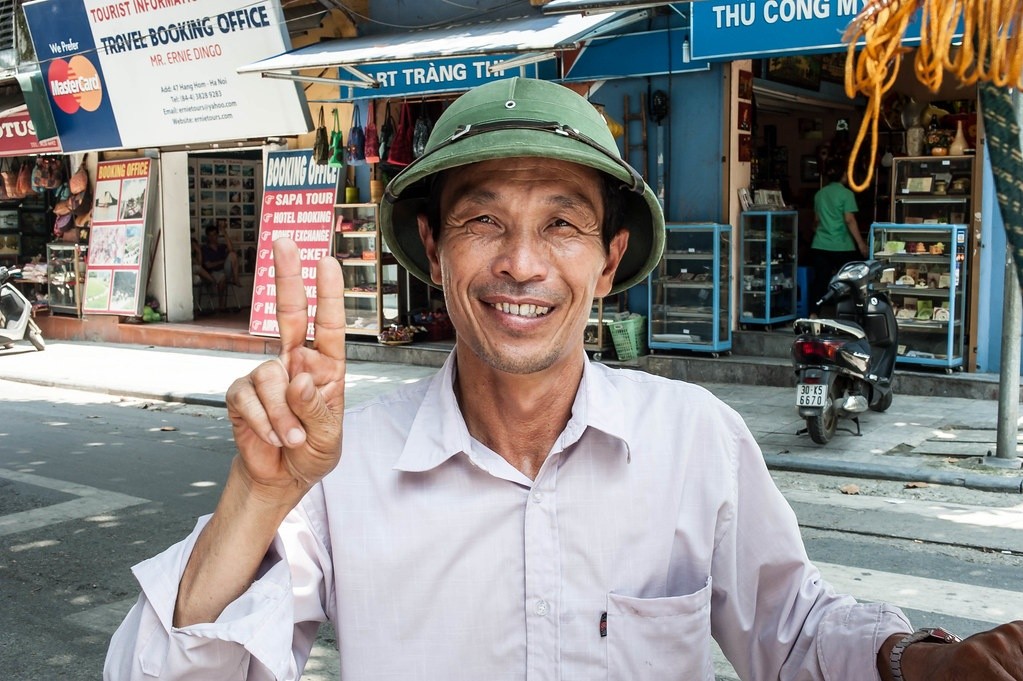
[195,279,242,314]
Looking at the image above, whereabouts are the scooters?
[0,264,46,351]
[789,257,899,445]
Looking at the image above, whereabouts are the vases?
[931,146,949,156]
[906,117,925,157]
[949,120,969,155]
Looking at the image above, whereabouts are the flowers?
[923,129,955,148]
[948,100,973,125]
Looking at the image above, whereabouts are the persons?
[100,77,1023,681]
[809,158,868,319]
[191,221,243,296]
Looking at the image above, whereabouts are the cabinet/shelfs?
[584,293,621,352]
[865,222,970,373]
[647,220,732,353]
[737,210,800,328]
[333,203,411,341]
[890,153,975,242]
[46,242,88,318]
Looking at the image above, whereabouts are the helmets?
[378,77,665,298]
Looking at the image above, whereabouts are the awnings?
[237,11,658,88]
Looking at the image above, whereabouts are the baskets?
[608,314,648,361]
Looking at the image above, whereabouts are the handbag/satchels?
[314,100,433,168]
[0,156,94,242]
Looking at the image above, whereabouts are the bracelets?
[889,626,963,681]
